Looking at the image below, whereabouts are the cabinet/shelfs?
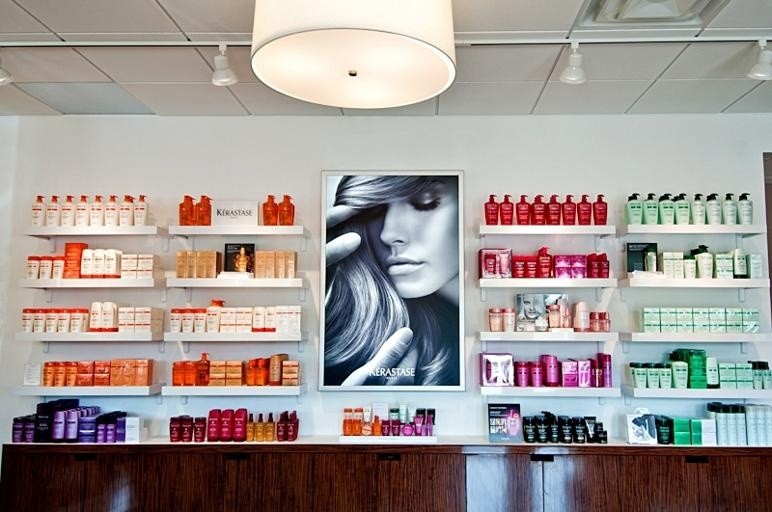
[161,225,310,404]
[481,224,622,405]
[622,224,772,404]
[18,225,168,404]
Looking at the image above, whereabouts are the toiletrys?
[26,350,300,386]
[23,242,299,276]
[343,405,436,437]
[487,302,612,332]
[522,415,585,443]
[639,307,762,333]
[627,346,771,389]
[627,401,771,447]
[484,195,754,225]
[478,241,765,278]
[13,395,301,440]
[24,192,301,226]
[516,353,613,388]
[21,299,300,332]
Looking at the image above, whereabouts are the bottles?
[343,404,435,436]
[488,300,612,333]
[171,360,196,387]
[523,411,585,443]
[511,253,609,278]
[629,350,772,445]
[246,412,276,441]
[645,248,696,279]
[277,410,300,441]
[245,356,271,385]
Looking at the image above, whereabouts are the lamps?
[251,1,456,111]
[0,29,772,88]
